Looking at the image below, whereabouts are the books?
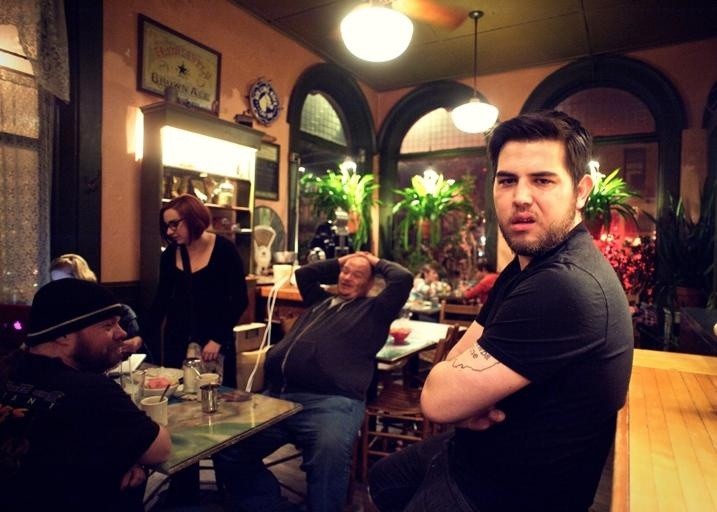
[105,351,148,378]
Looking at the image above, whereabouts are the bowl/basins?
[141,384,178,402]
[272,251,296,264]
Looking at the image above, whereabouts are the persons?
[363,110,635,511]
[209,250,413,511]
[48,253,138,340]
[303,208,371,267]
[0,276,171,511]
[120,192,249,511]
[406,223,503,311]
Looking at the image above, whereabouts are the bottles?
[182,343,203,394]
[118,344,134,401]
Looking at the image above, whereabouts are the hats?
[25,277,122,343]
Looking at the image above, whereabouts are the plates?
[107,353,147,375]
[143,367,184,382]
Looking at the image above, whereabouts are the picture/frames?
[253,138,280,203]
[133,11,222,120]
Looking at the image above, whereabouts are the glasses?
[161,219,183,230]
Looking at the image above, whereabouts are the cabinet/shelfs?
[139,101,266,278]
[232,276,259,324]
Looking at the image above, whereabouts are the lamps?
[449,10,498,134]
[338,1,414,65]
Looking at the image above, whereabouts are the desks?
[257,284,338,334]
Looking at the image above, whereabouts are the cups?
[141,395,170,429]
[129,369,146,405]
[195,373,221,403]
[200,384,219,415]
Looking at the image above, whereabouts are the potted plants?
[386,169,476,262]
[580,160,660,242]
[639,171,717,315]
[299,169,382,255]
